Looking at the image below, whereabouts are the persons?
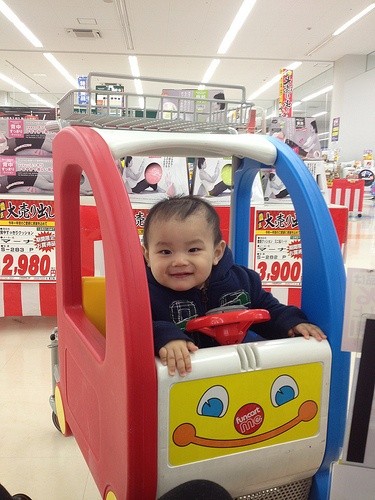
[285,120,318,157]
[198,158,233,197]
[0,169,54,194]
[3,129,54,156]
[122,155,166,193]
[143,192,328,378]
[269,173,289,198]
